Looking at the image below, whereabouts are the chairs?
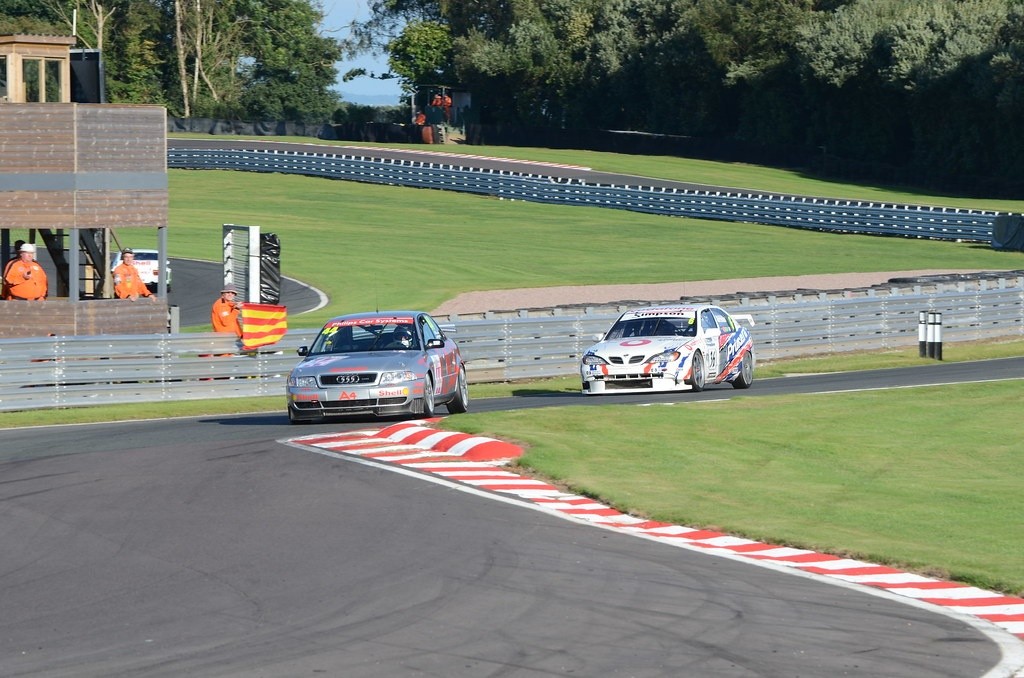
[338,335,360,350]
[373,332,393,349]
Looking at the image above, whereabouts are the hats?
[122,247,134,255]
[17,243,36,252]
[220,283,238,296]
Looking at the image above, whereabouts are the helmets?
[392,325,413,348]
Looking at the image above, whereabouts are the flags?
[241,301,288,352]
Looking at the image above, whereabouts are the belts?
[10,295,41,300]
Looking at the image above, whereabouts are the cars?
[113,249,172,293]
[578,301,755,399]
[287,308,469,422]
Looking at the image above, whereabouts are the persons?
[442,92,453,125]
[432,91,444,107]
[1,240,39,299]
[393,324,414,348]
[2,242,49,302]
[212,282,243,380]
[112,247,157,304]
[416,109,427,126]
